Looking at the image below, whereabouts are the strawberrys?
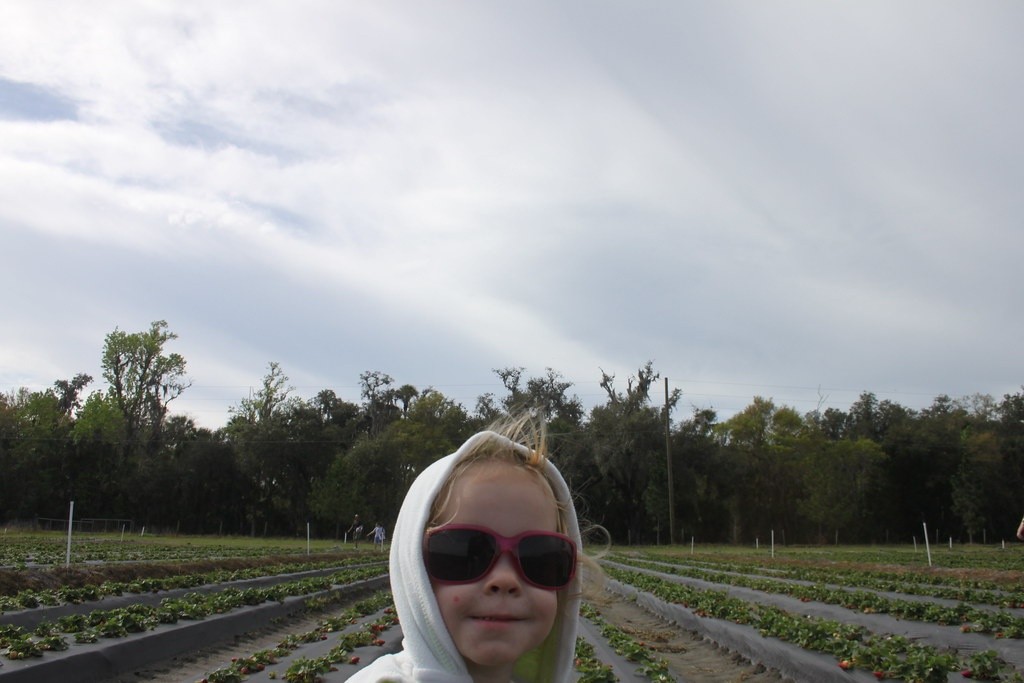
[197,607,398,683]
[571,598,1006,680]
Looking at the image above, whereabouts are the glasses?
[423,523,577,590]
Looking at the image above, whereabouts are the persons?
[342,402,611,683]
[1017,518,1024,539]
[348,514,362,549]
[366,522,385,550]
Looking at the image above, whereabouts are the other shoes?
[353,547,359,550]
